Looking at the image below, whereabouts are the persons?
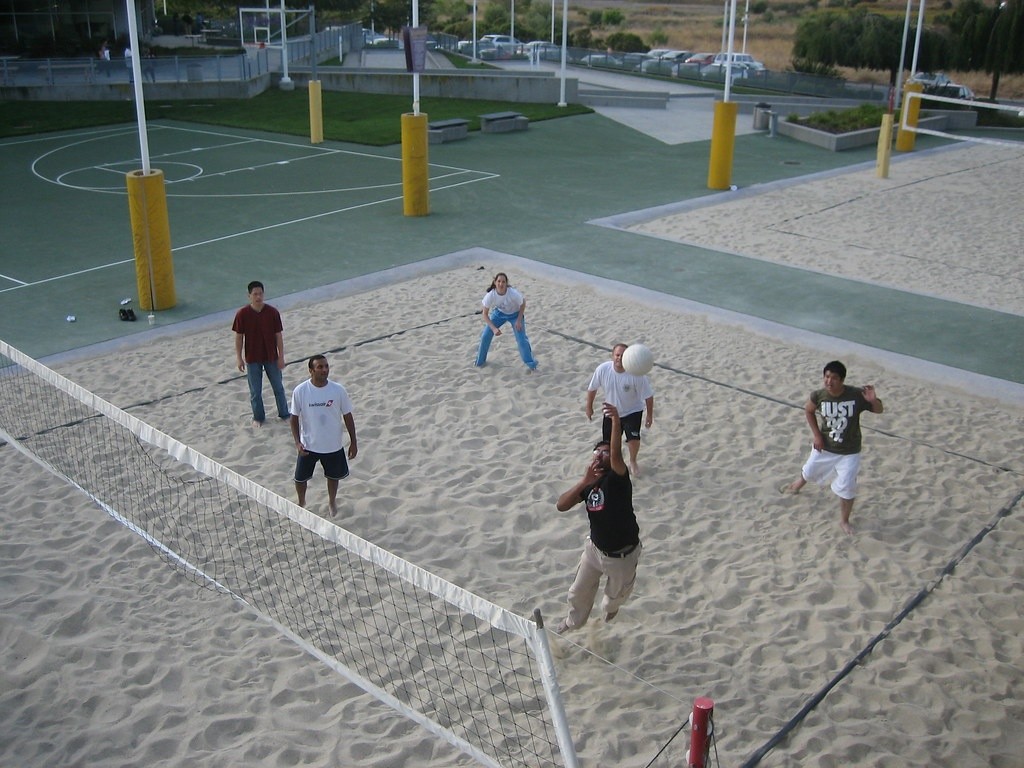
[586,344,654,474]
[195,12,204,34]
[290,355,357,518]
[124,42,134,83]
[182,10,193,39]
[96,39,112,78]
[790,360,884,536]
[143,48,157,83]
[232,281,292,423]
[172,11,181,36]
[476,272,539,370]
[557,401,643,634]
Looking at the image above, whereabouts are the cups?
[148,315,155,325]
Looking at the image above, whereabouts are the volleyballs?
[621,342,656,377]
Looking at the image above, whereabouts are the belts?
[601,543,637,558]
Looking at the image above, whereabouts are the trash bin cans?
[187,63,203,81]
[752,102,771,129]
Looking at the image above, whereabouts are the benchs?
[427,118,470,144]
[478,111,529,131]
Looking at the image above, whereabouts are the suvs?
[715,53,764,74]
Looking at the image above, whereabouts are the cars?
[480,35,525,49]
[941,84,974,101]
[672,62,703,79]
[619,52,652,67]
[661,50,693,64]
[363,28,385,42]
[452,39,495,55]
[648,49,669,58]
[685,53,716,65]
[904,72,945,91]
[524,41,560,57]
[581,54,616,67]
[700,64,748,81]
[634,59,676,77]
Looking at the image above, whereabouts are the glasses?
[592,449,611,457]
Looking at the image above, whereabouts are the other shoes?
[119,309,129,320]
[127,309,137,321]
